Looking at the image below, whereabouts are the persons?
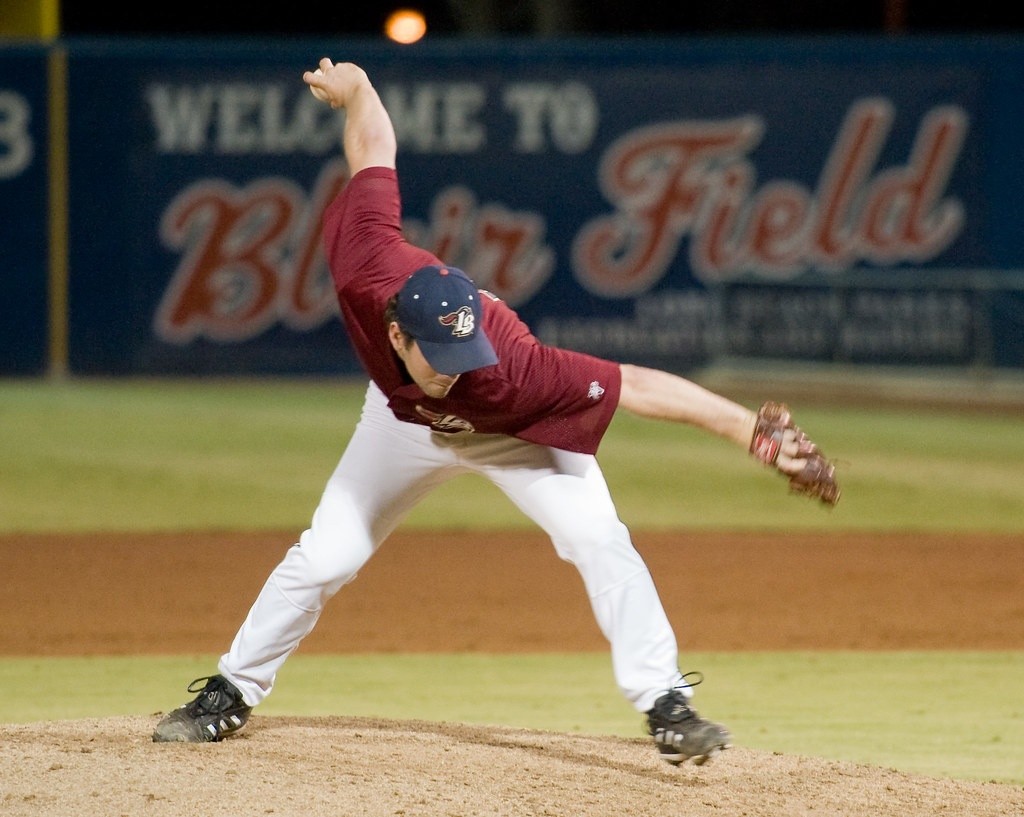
[153,58,841,762]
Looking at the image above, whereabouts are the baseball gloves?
[748,398,853,517]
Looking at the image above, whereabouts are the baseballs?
[308,68,330,102]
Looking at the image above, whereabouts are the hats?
[395,265,499,375]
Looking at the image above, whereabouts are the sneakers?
[152,674,254,743]
[643,672,729,761]
